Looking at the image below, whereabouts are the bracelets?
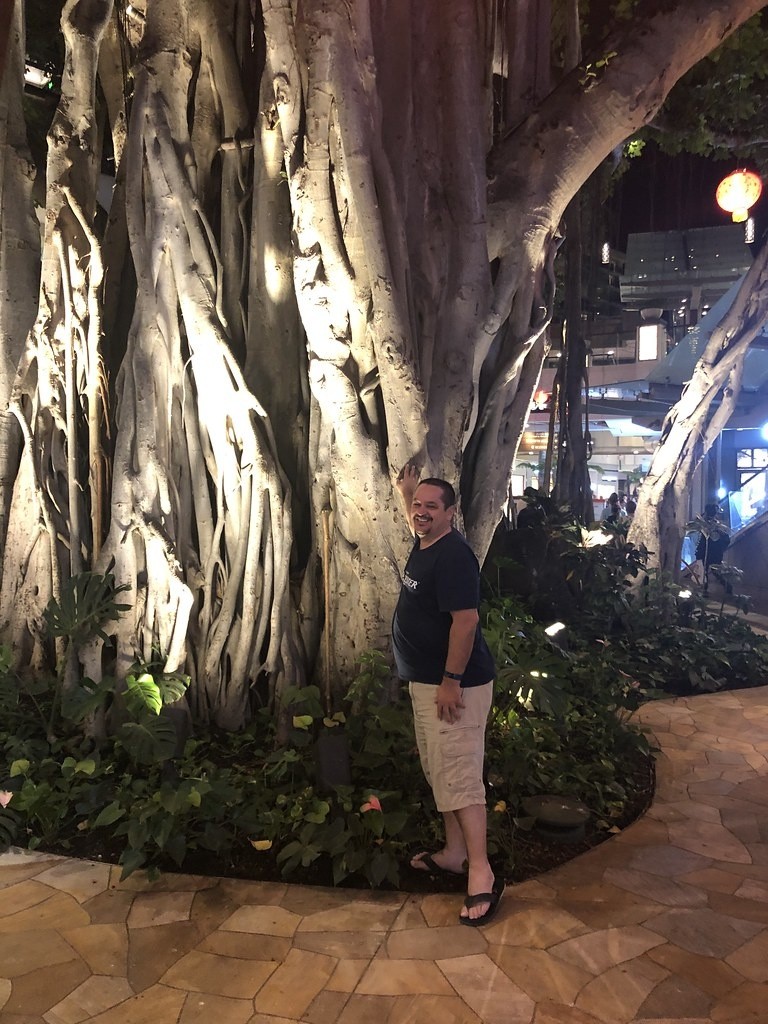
[443,671,464,680]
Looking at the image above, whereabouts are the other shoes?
[725,584,733,596]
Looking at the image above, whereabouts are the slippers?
[406,851,468,879]
[459,878,506,929]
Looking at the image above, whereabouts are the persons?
[696,495,733,597]
[391,464,506,927]
[517,487,533,514]
[600,489,638,549]
